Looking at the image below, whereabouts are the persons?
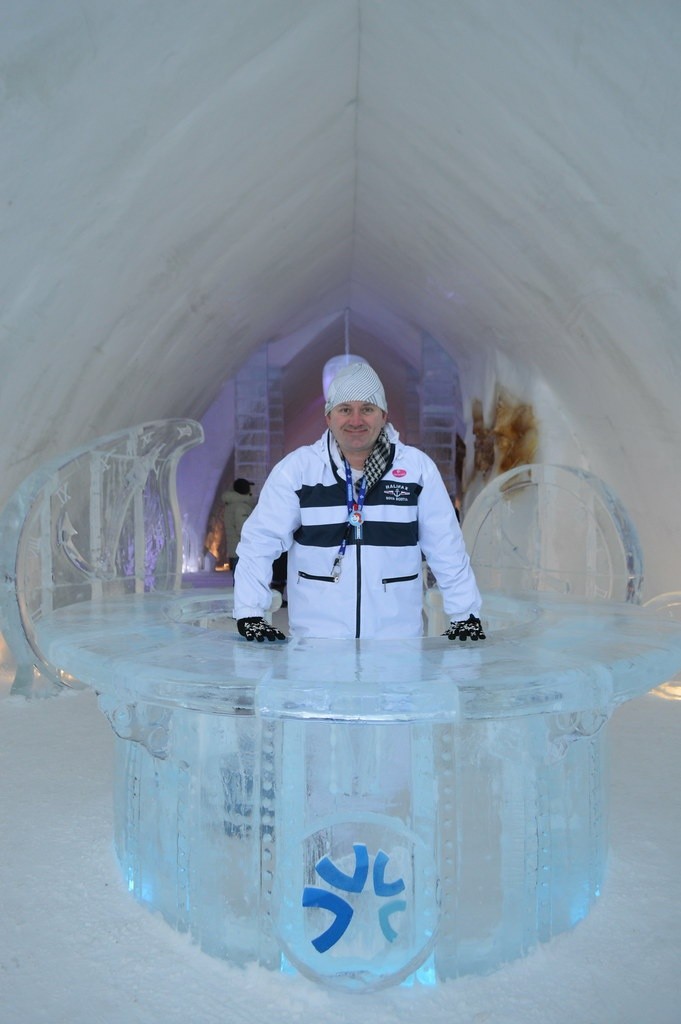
[231,364,486,641]
[222,478,256,588]
[268,550,288,608]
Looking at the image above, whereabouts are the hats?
[324,364,388,417]
[234,478,255,488]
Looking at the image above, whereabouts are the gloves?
[238,618,286,641]
[440,614,486,641]
